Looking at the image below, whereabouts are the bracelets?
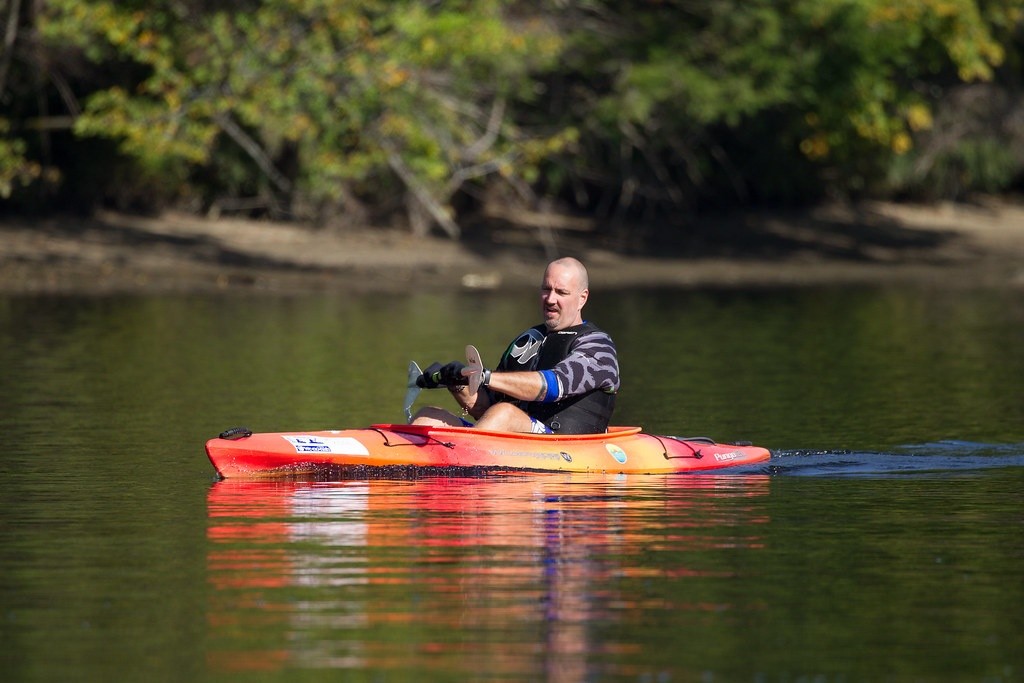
[483,368,491,389]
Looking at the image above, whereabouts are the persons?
[410,257,620,436]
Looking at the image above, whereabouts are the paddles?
[399,344,482,411]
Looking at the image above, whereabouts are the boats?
[205,421,775,477]
